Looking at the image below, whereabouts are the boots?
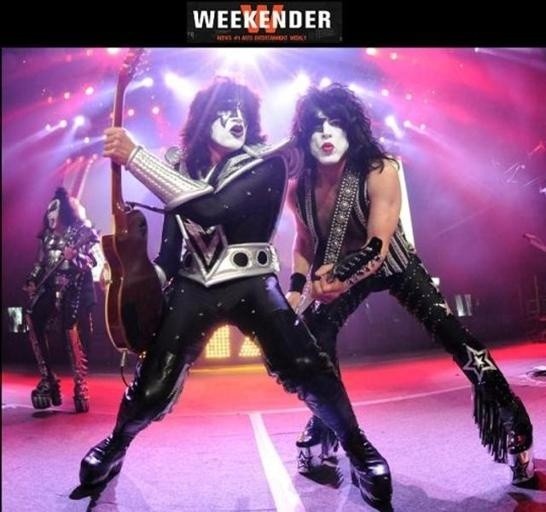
[66,329,89,413]
[294,371,394,506]
[77,386,157,486]
[25,325,61,409]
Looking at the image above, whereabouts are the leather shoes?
[297,413,341,476]
[500,408,536,485]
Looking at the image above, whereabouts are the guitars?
[100,45,164,360]
[21,231,96,309]
[297,237,382,325]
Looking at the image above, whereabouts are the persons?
[20,185,101,411]
[283,81,537,489]
[69,76,392,512]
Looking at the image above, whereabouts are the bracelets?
[287,273,305,295]
